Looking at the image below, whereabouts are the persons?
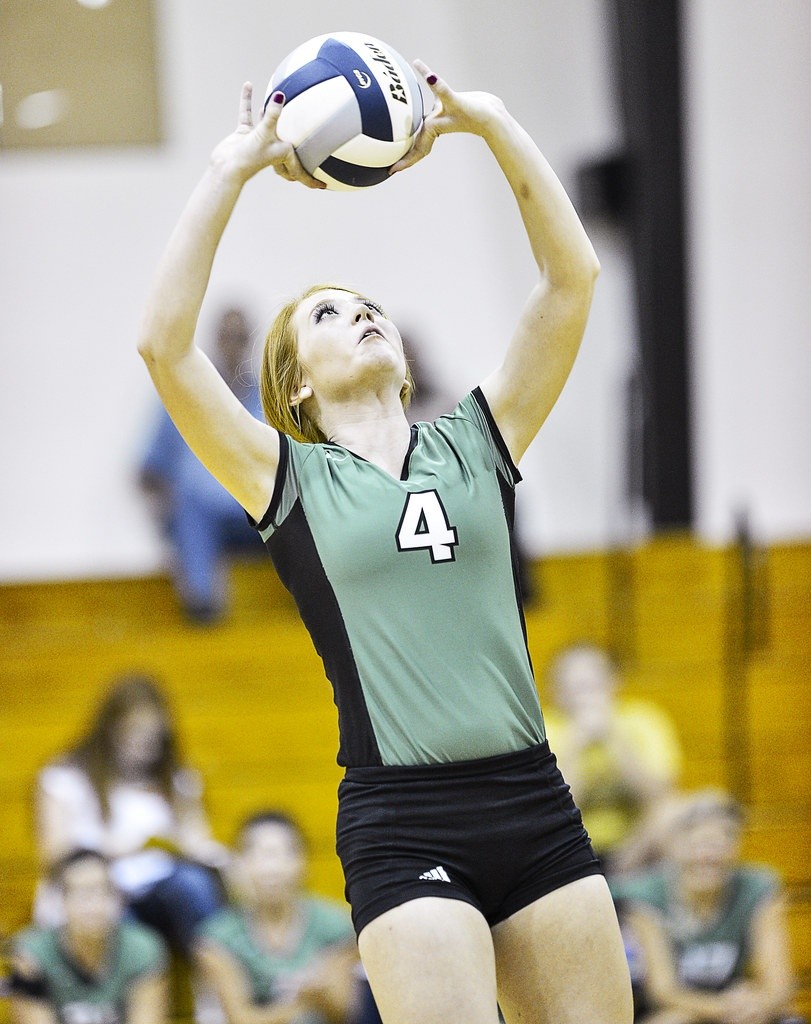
[142,308,268,631]
[136,60,635,1024]
[0,637,794,1024]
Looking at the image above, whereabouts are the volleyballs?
[263,28,424,190]
